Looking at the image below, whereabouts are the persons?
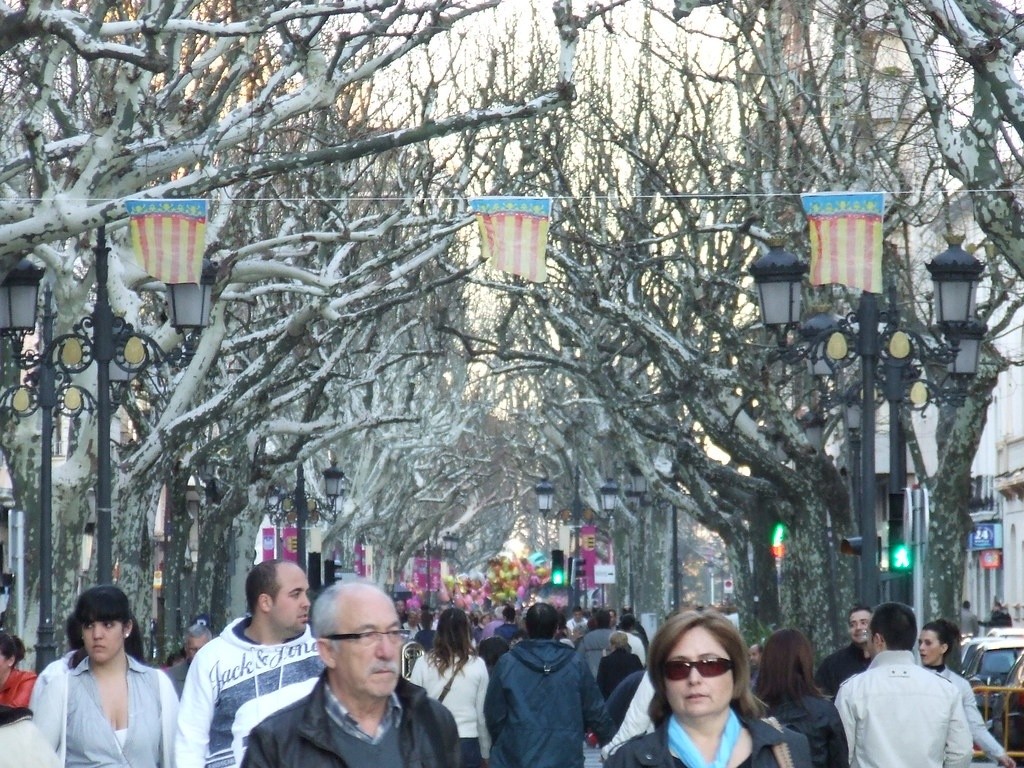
[2,559,1015,767]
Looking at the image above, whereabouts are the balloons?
[441,554,548,613]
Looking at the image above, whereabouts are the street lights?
[263,455,345,576]
[745,228,987,607]
[535,462,619,605]
[625,446,681,613]
[1,218,220,674]
[412,531,460,604]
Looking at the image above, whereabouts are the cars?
[958,627,1024,761]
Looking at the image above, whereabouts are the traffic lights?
[842,535,880,554]
[571,557,587,588]
[892,543,912,572]
[770,523,787,548]
[551,549,565,585]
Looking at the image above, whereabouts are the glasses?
[324,629,409,645]
[664,659,732,680]
